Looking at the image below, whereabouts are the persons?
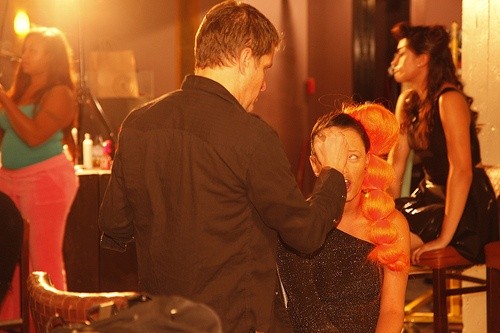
[0,191,24,309]
[390,22,495,272]
[0,28,80,333]
[274,111,410,333]
[97,1,350,333]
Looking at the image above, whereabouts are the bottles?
[82,133,93,169]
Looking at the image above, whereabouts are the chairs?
[26,271,150,333]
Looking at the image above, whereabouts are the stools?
[404,240,500,333]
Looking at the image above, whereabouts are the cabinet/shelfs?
[63,170,138,293]
